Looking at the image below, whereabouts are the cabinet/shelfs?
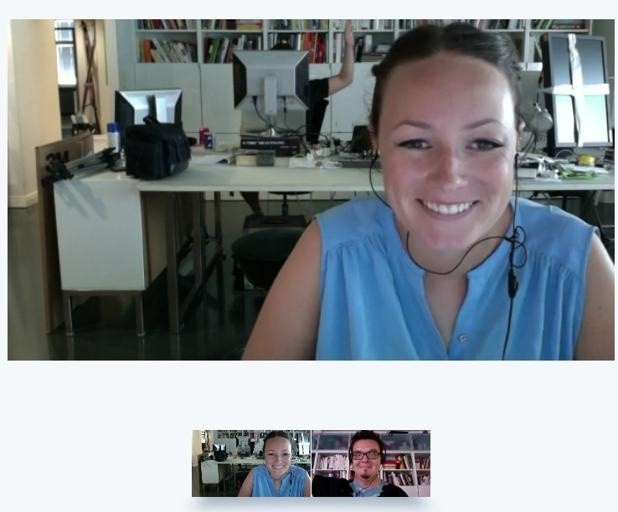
[115,19,594,153]
[53,167,205,336]
[201,460,229,487]
[213,430,310,458]
[312,431,430,497]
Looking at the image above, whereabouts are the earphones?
[370,149,380,169]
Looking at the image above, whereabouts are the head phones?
[349,438,386,464]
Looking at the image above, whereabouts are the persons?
[234,21,614,360]
[238,20,354,211]
[314,430,409,496]
[237,430,312,497]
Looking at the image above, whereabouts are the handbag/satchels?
[215,449,228,462]
[124,115,191,180]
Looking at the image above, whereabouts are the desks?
[216,457,310,480]
[137,153,614,334]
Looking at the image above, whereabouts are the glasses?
[350,451,381,460]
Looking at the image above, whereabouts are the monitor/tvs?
[540,32,613,148]
[297,433,310,456]
[213,443,226,452]
[232,50,309,116]
[236,436,251,449]
[114,89,182,147]
